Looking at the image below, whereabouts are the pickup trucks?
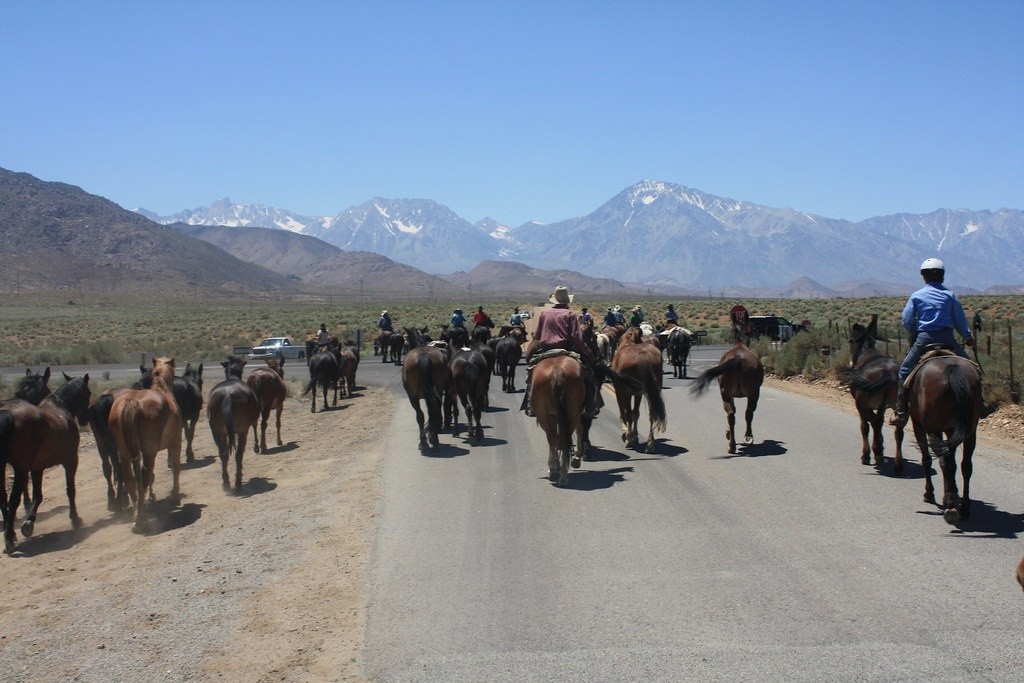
[747,315,808,343]
[248,336,307,360]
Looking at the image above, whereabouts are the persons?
[889,258,975,426]
[602,307,615,330]
[664,304,679,325]
[525,286,605,416]
[474,306,488,327]
[451,308,467,328]
[613,306,626,327]
[510,308,529,342]
[379,310,392,331]
[629,305,644,326]
[312,323,329,353]
[973,311,983,332]
[578,306,591,324]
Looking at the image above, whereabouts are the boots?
[889,378,909,427]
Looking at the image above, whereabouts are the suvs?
[521,311,530,319]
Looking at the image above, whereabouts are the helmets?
[919,257,946,276]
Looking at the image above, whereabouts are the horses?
[206,355,261,496]
[689,310,764,454]
[906,317,981,521]
[835,315,909,473]
[525,319,626,484]
[247,357,286,454]
[89,356,181,534]
[402,324,528,455]
[303,336,360,413]
[641,322,694,379]
[167,362,203,468]
[612,328,667,453]
[373,326,433,366]
[0,366,92,555]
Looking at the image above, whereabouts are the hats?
[581,306,588,311]
[614,305,621,312]
[667,304,674,309]
[512,308,520,314]
[477,306,484,310]
[636,305,641,310]
[454,308,463,314]
[606,307,613,312]
[381,310,388,316]
[548,285,574,305]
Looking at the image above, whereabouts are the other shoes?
[526,408,534,416]
[584,408,599,418]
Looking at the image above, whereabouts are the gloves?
[964,332,976,347]
